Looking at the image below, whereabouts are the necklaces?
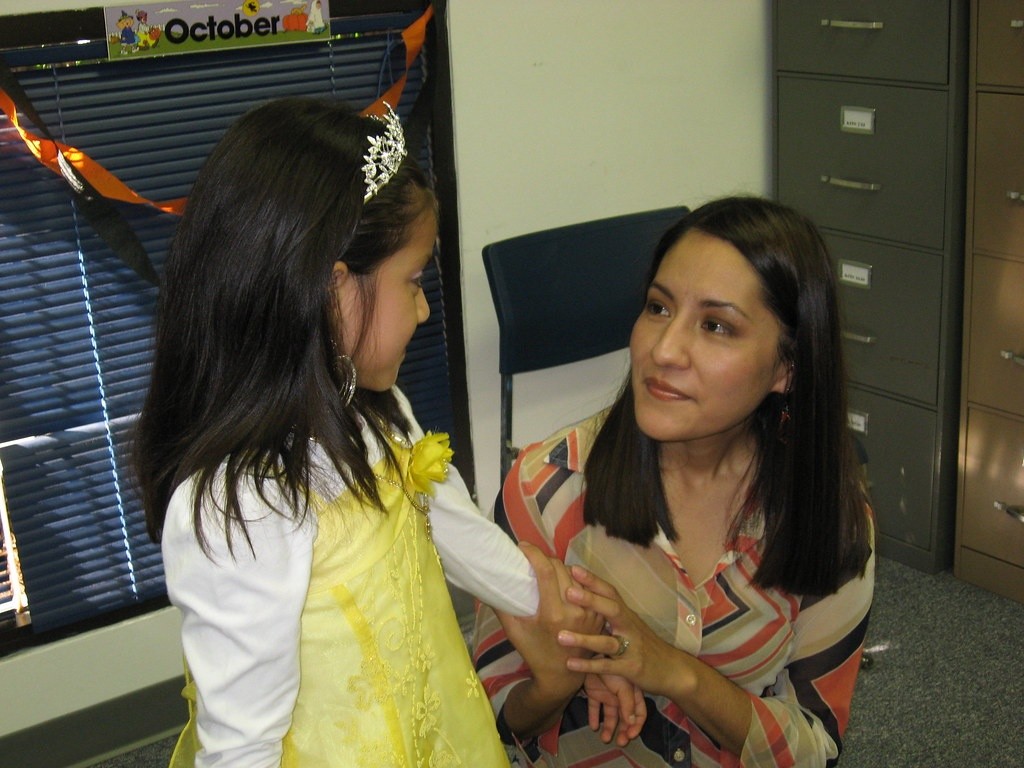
[375,474,432,539]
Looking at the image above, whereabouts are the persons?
[473,195,880,768]
[122,93,647,768]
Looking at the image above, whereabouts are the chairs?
[482,206,691,483]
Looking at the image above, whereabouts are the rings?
[612,634,630,656]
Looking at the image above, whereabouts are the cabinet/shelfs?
[770,0,969,575]
[954,0,1024,603]
